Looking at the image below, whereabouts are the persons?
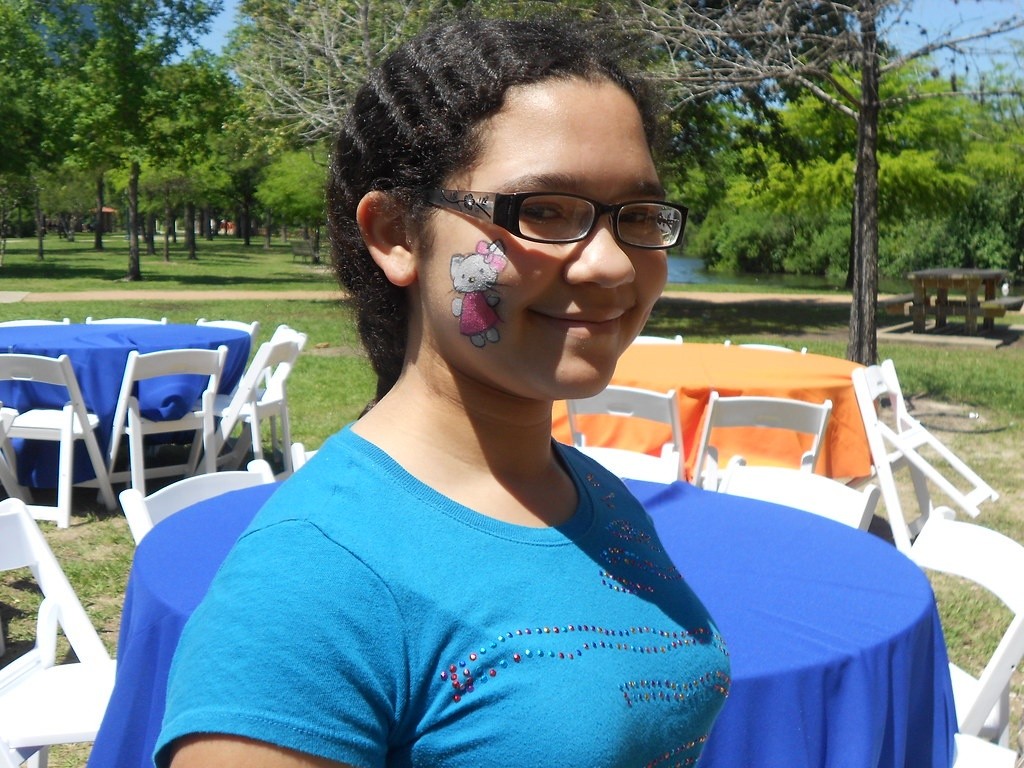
[151,2,730,768]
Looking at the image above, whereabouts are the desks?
[0,324,250,488]
[902,267,1007,337]
[81,468,960,767]
[548,340,878,483]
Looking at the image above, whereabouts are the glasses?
[427,187,691,249]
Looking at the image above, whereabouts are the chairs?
[1,317,1024,768]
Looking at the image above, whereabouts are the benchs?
[878,291,932,316]
[977,295,1024,318]
